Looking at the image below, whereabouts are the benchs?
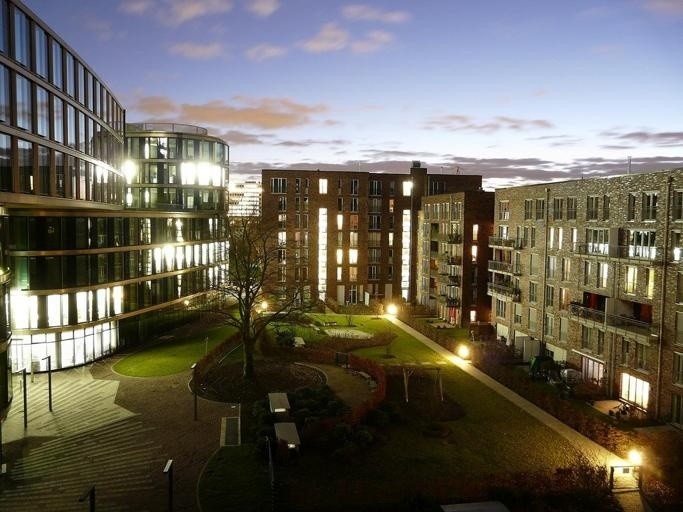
[358,371,372,380]
